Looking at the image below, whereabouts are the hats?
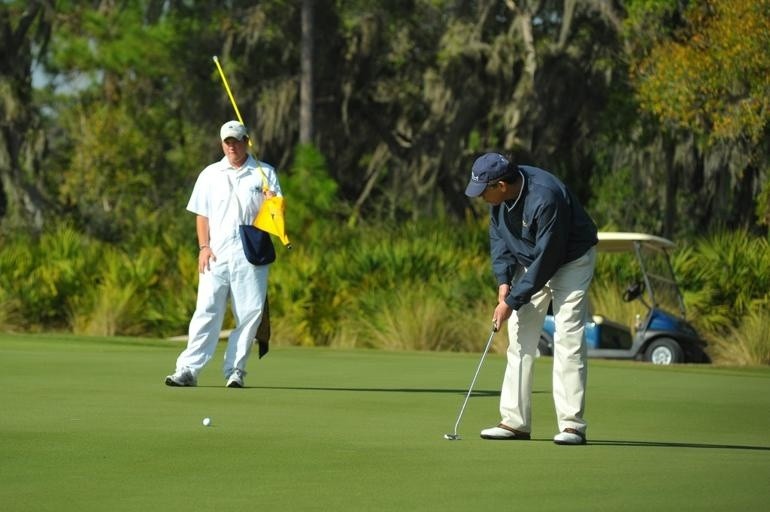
[464,152,513,197]
[220,120,249,141]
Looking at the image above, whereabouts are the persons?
[164,120,285,388]
[463,151,599,444]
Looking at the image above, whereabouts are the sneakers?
[480,423,530,440]
[226,369,244,387]
[553,427,587,445]
[165,368,197,386]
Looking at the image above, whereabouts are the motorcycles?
[515,231,713,365]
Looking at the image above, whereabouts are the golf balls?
[203,418,209,426]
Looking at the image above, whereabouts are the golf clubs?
[444,320,497,439]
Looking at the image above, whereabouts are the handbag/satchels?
[239,224,276,265]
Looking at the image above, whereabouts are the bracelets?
[198,246,210,251]
